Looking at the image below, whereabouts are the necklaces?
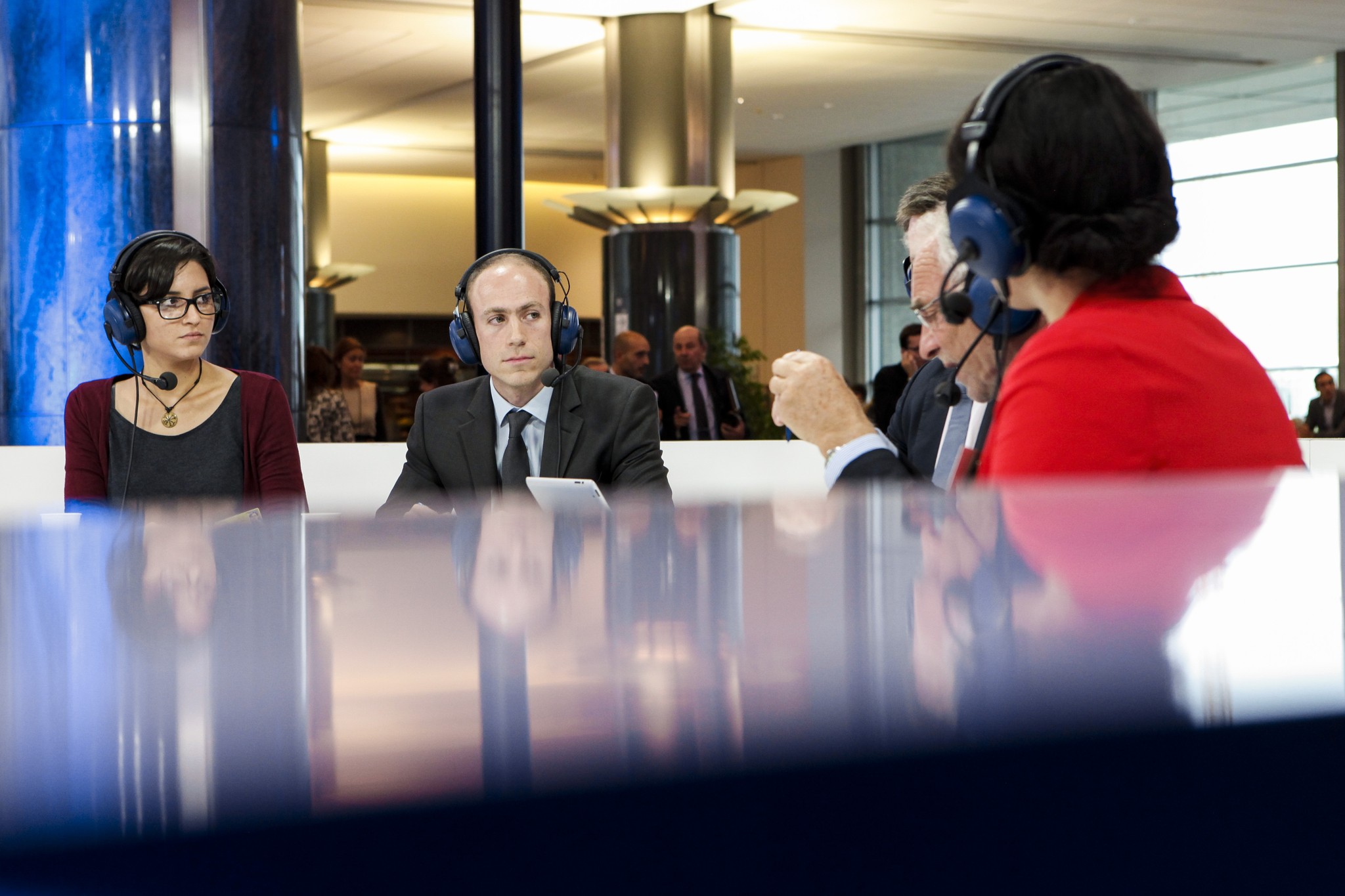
[141,356,203,428]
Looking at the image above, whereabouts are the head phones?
[449,248,582,365]
[904,173,1038,344]
[950,52,1095,277]
[103,230,232,344]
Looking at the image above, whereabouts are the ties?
[930,385,972,494]
[686,372,713,440]
[502,411,535,504]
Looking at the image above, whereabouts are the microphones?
[102,320,177,391]
[938,299,1005,406]
[543,325,583,385]
[940,238,981,327]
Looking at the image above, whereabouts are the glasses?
[142,291,222,320]
[912,275,966,330]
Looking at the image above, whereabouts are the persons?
[451,515,586,636]
[946,51,1302,478]
[301,345,355,441]
[334,336,389,441]
[949,470,1289,743]
[416,352,459,396]
[102,525,245,650]
[874,170,989,492]
[870,323,929,431]
[1295,368,1344,439]
[652,324,752,441]
[602,329,663,440]
[372,241,678,514]
[62,227,315,519]
[766,198,1049,490]
[909,497,1057,719]
